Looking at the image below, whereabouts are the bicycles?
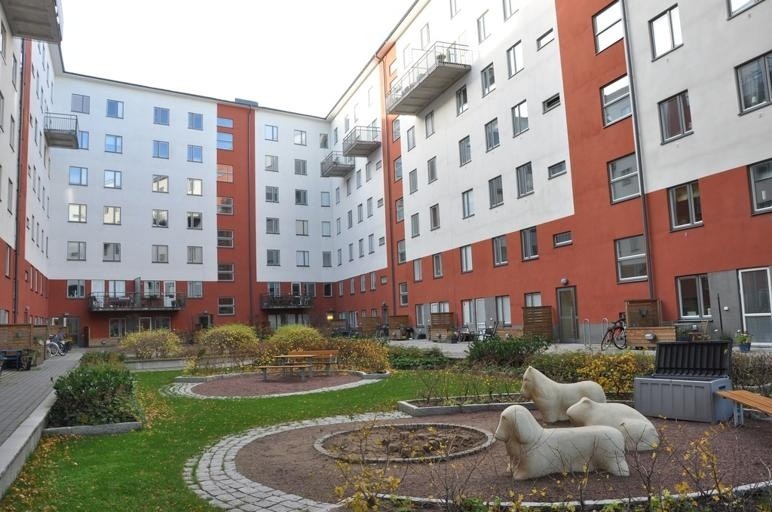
[600,318,626,351]
[46,332,73,357]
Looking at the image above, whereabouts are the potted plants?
[736,332,750,353]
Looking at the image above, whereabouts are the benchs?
[257,348,341,381]
[715,386,771,430]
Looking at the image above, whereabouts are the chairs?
[459,321,499,343]
